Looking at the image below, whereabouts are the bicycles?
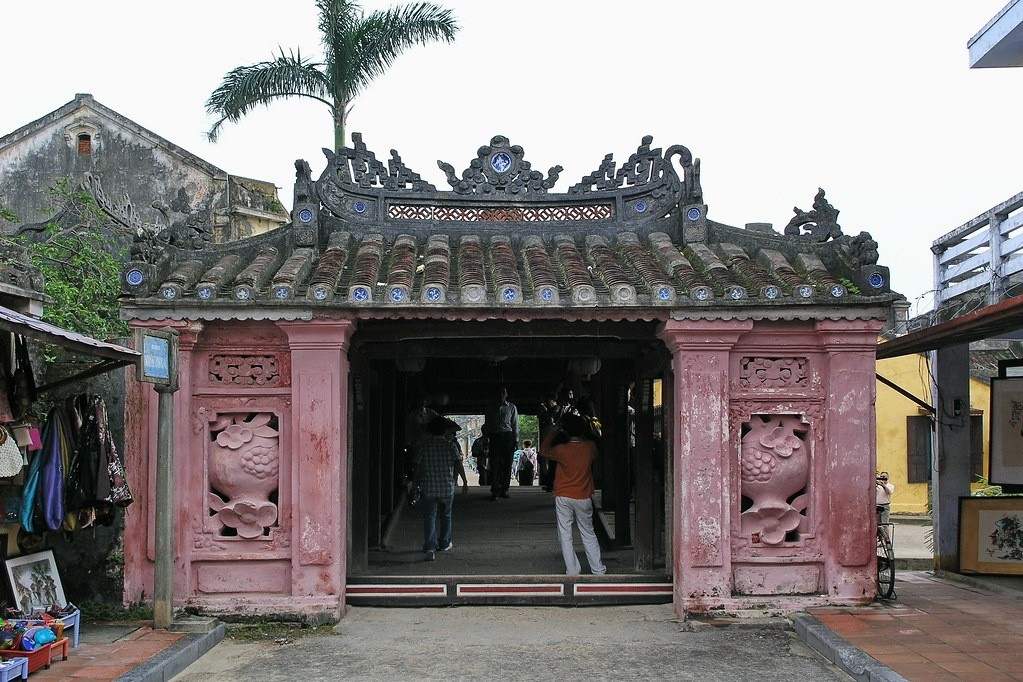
[877,502,896,599]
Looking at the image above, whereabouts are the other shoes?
[492,490,509,501]
[887,543,892,551]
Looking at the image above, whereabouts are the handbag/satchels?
[0,422,43,478]
[0,364,37,421]
[61,506,109,543]
[32,498,50,534]
[0,484,25,523]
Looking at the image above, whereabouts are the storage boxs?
[0,606,80,682]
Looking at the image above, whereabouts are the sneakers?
[593,565,606,575]
[565,571,568,575]
[424,550,435,561]
[440,541,453,551]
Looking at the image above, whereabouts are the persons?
[404,384,539,501]
[409,417,468,560]
[875,472,894,547]
[539,413,607,576]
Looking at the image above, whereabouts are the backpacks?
[521,452,535,476]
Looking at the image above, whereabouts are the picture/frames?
[957,496,1023,577]
[990,378,1023,493]
[5,547,69,620]
[999,360,1023,493]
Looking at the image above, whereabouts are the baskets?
[0,636,69,682]
[9,610,80,647]
